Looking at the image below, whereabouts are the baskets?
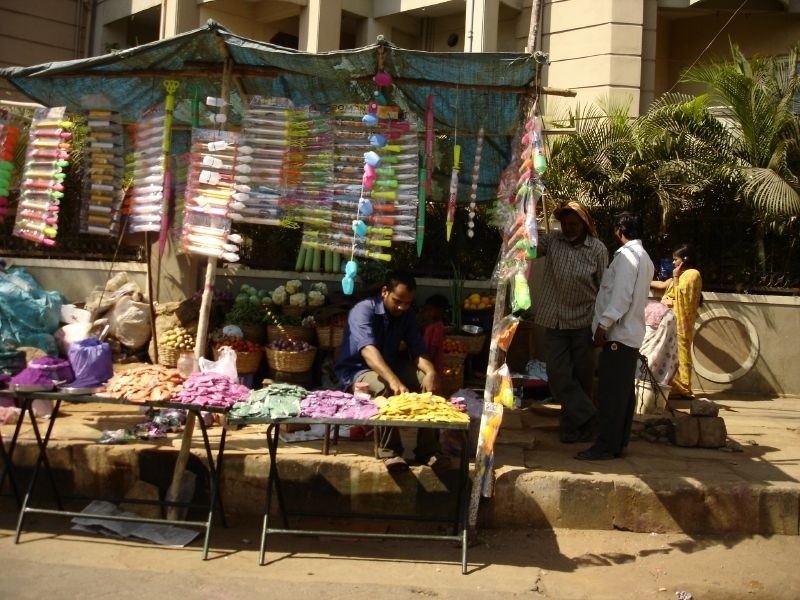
[445,335,486,352]
[440,356,464,392]
[157,318,346,371]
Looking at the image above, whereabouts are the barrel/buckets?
[634,377,672,414]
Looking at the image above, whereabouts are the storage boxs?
[635,379,673,418]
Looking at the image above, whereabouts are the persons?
[572,213,655,460]
[651,245,703,401]
[531,200,607,441]
[330,270,451,468]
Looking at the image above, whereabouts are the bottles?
[517,385,525,408]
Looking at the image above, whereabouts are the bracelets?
[673,276,679,278]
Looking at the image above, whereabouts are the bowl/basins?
[461,324,483,336]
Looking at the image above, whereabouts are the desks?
[0,382,235,561]
[227,391,473,574]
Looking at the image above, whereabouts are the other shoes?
[575,443,622,461]
[670,392,695,400]
[385,456,409,473]
[560,415,599,444]
[417,449,451,468]
